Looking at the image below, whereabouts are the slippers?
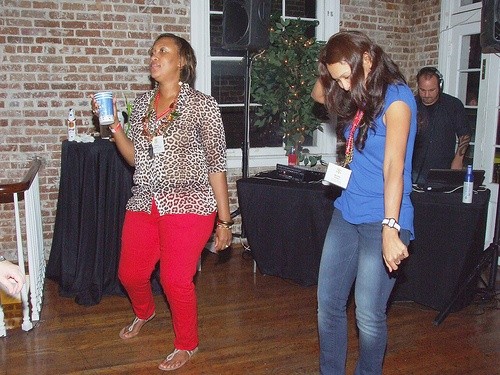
[158,345,199,370]
[118,309,155,339]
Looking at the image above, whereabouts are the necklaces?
[143,90,181,140]
[156,92,172,120]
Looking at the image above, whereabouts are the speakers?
[480,0,500,54]
[221,0,272,51]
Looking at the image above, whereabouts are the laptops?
[411,169,485,192]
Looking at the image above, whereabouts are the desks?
[45,138,178,307]
[236,171,490,312]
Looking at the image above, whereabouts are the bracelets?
[218,219,235,225]
[217,222,232,229]
[109,124,121,133]
[382,217,400,232]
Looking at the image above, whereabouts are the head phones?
[417,67,444,93]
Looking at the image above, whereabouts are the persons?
[411,67,472,184]
[311,30,416,375]
[91,33,233,371]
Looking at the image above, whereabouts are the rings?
[226,244,230,247]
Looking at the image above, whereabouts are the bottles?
[461,165,474,203]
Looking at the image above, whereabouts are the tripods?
[432,187,500,327]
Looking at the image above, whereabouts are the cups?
[93,92,114,125]
